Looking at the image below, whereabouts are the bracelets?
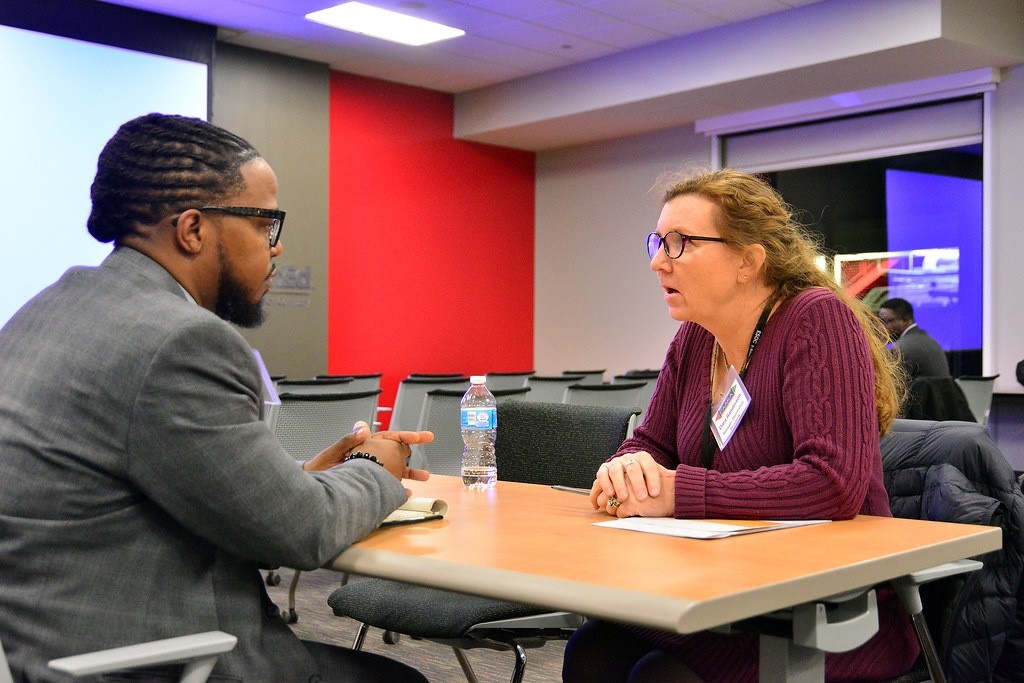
[345,451,384,467]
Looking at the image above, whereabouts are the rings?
[608,496,620,508]
[626,460,636,467]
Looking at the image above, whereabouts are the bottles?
[459,376,498,489]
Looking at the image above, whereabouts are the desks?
[317,471,1005,683]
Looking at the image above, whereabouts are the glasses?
[171,209,286,245]
[646,229,730,259]
[881,316,899,325]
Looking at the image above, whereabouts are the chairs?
[407,373,464,381]
[563,369,607,385]
[407,386,534,472]
[316,373,393,433]
[881,419,992,683]
[271,387,383,625]
[525,376,588,404]
[957,374,1001,425]
[0,630,238,683]
[904,376,954,419]
[485,371,536,401]
[274,378,356,394]
[389,377,471,463]
[325,400,642,683]
[565,381,648,435]
[614,374,658,426]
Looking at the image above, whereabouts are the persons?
[563,171,922,683]
[0,112,434,683]
[878,298,950,402]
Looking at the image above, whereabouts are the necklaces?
[723,351,729,371]
